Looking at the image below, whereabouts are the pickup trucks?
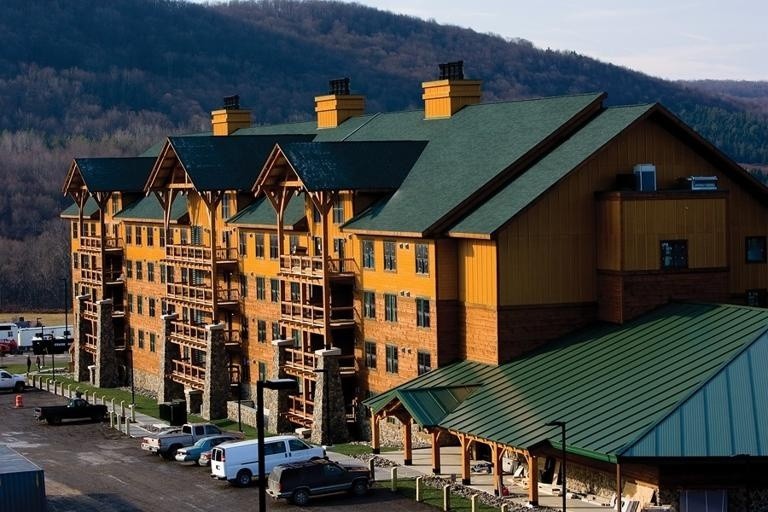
[34,398,108,428]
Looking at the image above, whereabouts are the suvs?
[266,457,371,507]
[0,369,28,392]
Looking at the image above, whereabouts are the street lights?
[313,368,333,445]
[59,278,68,351]
[114,347,134,405]
[41,325,45,365]
[42,333,55,380]
[225,364,242,432]
[544,420,567,512]
[255,377,297,511]
[36,318,42,327]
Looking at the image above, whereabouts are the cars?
[141,422,245,467]
[0,338,17,355]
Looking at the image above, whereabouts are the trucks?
[0,322,73,354]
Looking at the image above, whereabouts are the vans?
[209,434,326,486]
